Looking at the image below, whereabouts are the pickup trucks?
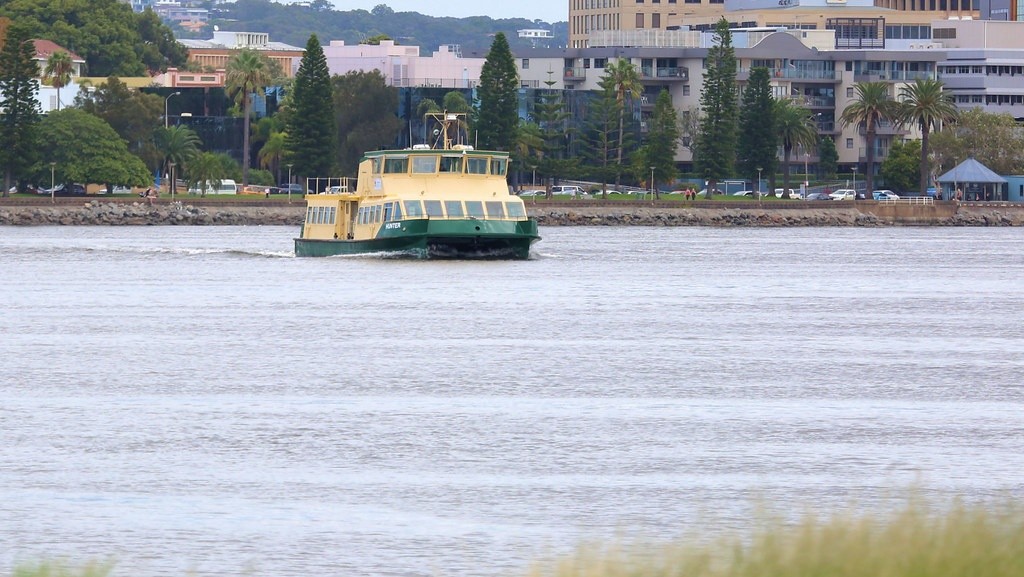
[320,186,354,195]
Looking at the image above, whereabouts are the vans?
[189,179,236,195]
[550,185,588,196]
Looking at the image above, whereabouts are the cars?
[514,189,547,197]
[803,189,901,201]
[629,190,723,196]
[734,189,806,200]
[270,183,313,194]
[595,191,624,195]
[10,185,38,194]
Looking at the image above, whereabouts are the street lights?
[49,162,57,203]
[165,92,181,130]
[851,167,858,201]
[170,163,177,198]
[650,167,656,201]
[284,164,294,205]
[756,167,764,201]
[530,165,539,204]
[804,112,821,194]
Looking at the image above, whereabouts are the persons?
[957,188,963,201]
[685,188,690,200]
[145,189,151,198]
[265,188,269,198]
[691,188,696,200]
[440,164,447,170]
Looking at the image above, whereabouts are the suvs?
[99,184,139,195]
[42,184,85,194]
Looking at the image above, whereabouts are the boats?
[292,108,542,258]
